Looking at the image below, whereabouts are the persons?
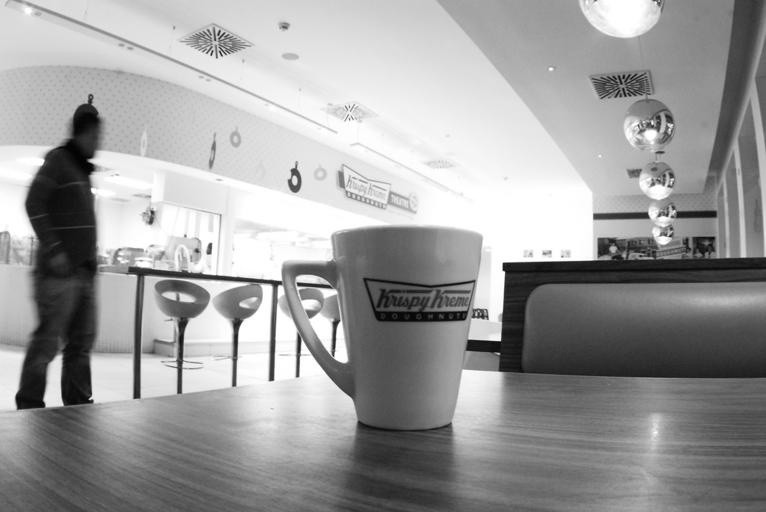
[14,112,101,409]
[608,243,622,257]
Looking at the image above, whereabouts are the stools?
[153,280,341,395]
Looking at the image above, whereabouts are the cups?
[281,227,483,432]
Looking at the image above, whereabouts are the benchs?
[496,255,764,376]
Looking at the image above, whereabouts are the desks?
[1,369,765,512]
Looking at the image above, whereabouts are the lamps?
[577,0,679,247]
